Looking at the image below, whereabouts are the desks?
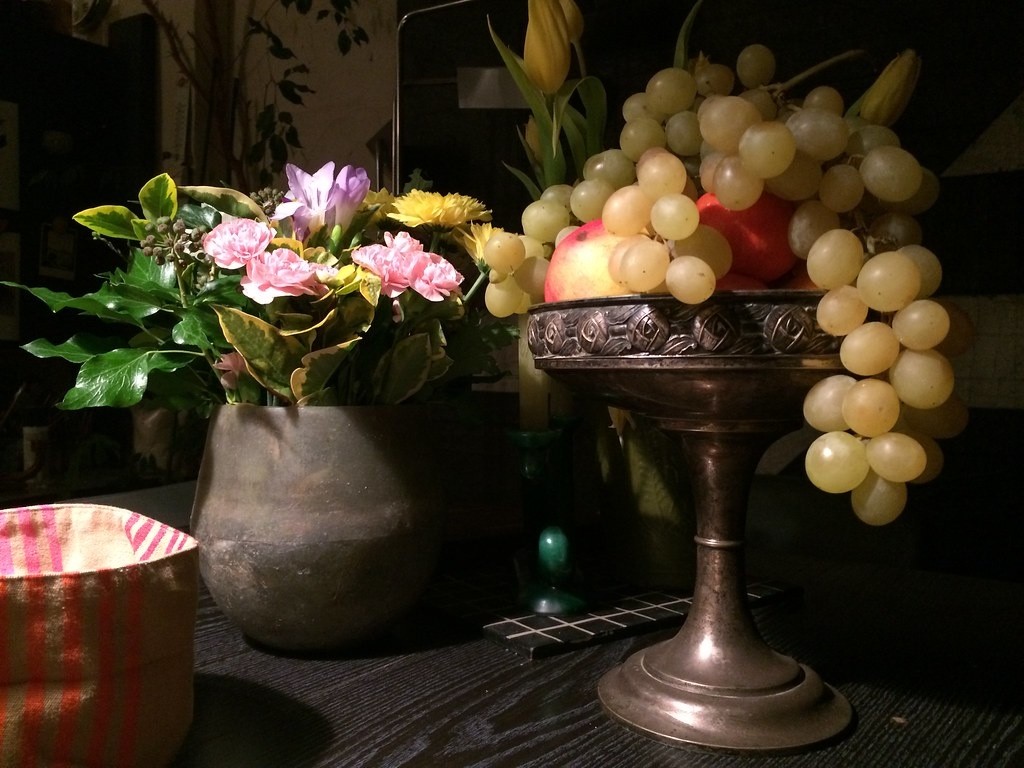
[0,478,1024,768]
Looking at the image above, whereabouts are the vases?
[193,402,452,650]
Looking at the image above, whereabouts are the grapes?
[485,41,965,526]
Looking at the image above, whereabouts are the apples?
[775,260,820,289]
[693,189,804,278]
[712,274,763,290]
[544,217,639,301]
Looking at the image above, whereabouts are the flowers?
[0,160,521,417]
